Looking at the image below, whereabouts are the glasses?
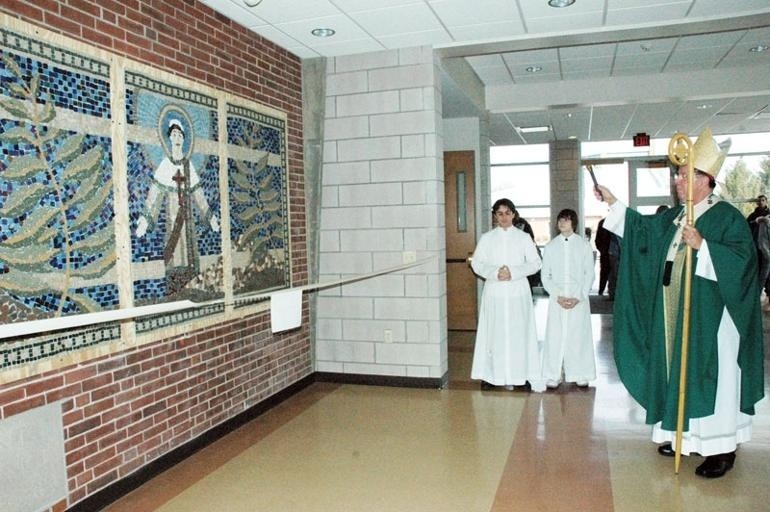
[674,172,702,185]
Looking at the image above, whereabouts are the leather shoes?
[659,443,700,456]
[694,453,736,478]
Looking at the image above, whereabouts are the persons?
[491,194,770,318]
[134,118,221,299]
[538,208,596,389]
[469,198,544,392]
[589,161,764,478]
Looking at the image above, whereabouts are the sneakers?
[481,380,589,393]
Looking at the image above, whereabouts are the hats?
[169,118,184,132]
[684,126,732,182]
